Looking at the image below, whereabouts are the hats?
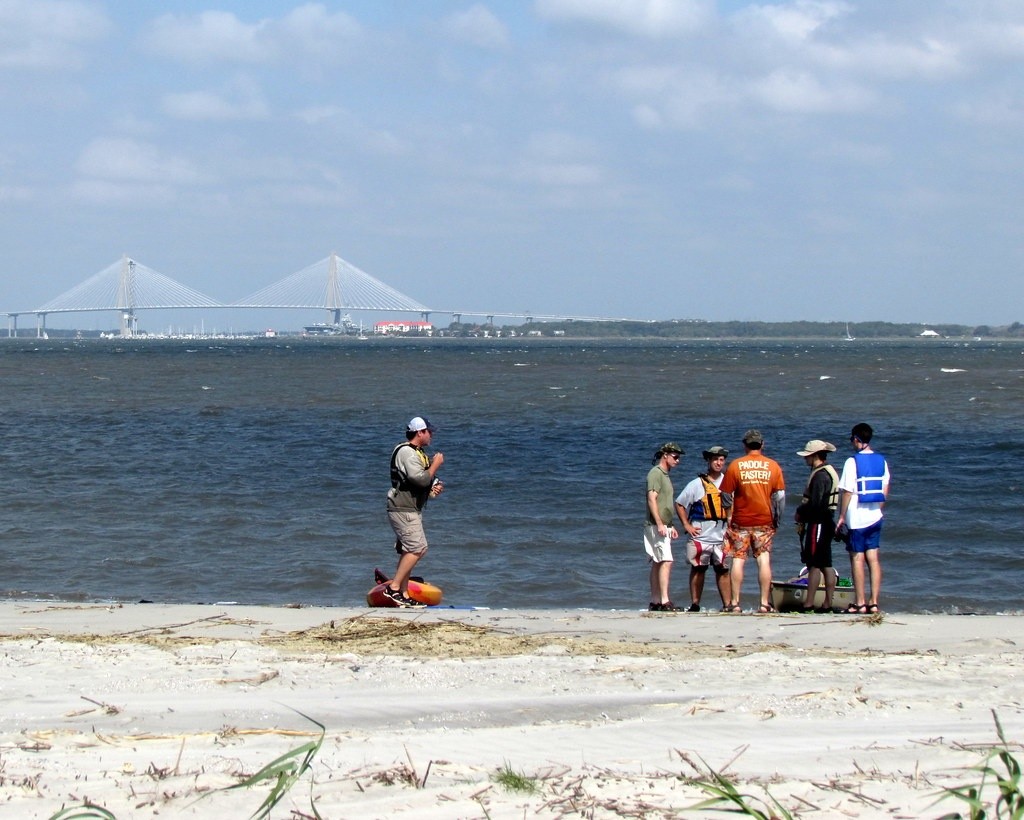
[407,417,438,432]
[743,429,761,444]
[702,446,729,461]
[795,440,836,457]
[660,442,685,454]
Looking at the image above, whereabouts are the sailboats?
[843,321,856,343]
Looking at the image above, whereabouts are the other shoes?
[798,605,814,613]
[688,605,700,612]
[659,601,684,611]
[649,602,660,611]
[814,605,834,614]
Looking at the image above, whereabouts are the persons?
[794,439,841,614]
[387,416,444,609]
[643,442,686,611]
[718,430,785,613]
[674,445,731,611]
[835,423,891,614]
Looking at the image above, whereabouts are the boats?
[365,567,444,606]
[766,564,856,612]
[939,366,968,374]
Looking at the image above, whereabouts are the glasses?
[850,436,861,443]
[667,453,679,460]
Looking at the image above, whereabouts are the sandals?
[720,603,742,613]
[867,604,878,614]
[841,603,867,614]
[399,598,428,609]
[383,585,411,607]
[760,605,779,613]
